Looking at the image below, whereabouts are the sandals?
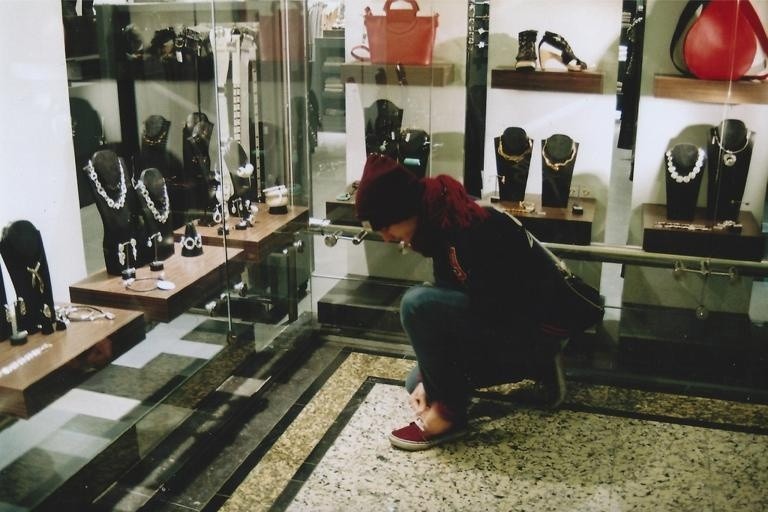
[538,30,588,73]
[515,29,538,70]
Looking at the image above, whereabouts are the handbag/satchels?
[350,0,438,65]
[669,0,768,82]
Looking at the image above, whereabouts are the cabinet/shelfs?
[314,0,768,395]
[2,2,311,512]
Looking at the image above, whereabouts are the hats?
[355,155,422,221]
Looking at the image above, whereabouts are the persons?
[355,153,603,455]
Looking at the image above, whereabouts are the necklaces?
[86,155,129,212]
[364,105,399,155]
[179,231,205,251]
[654,217,715,234]
[124,273,168,292]
[667,144,707,184]
[397,132,431,167]
[495,133,535,165]
[133,172,171,226]
[712,125,753,168]
[0,225,48,295]
[52,300,116,326]
[541,137,577,171]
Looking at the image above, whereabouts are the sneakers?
[389,412,468,451]
[535,350,566,411]
